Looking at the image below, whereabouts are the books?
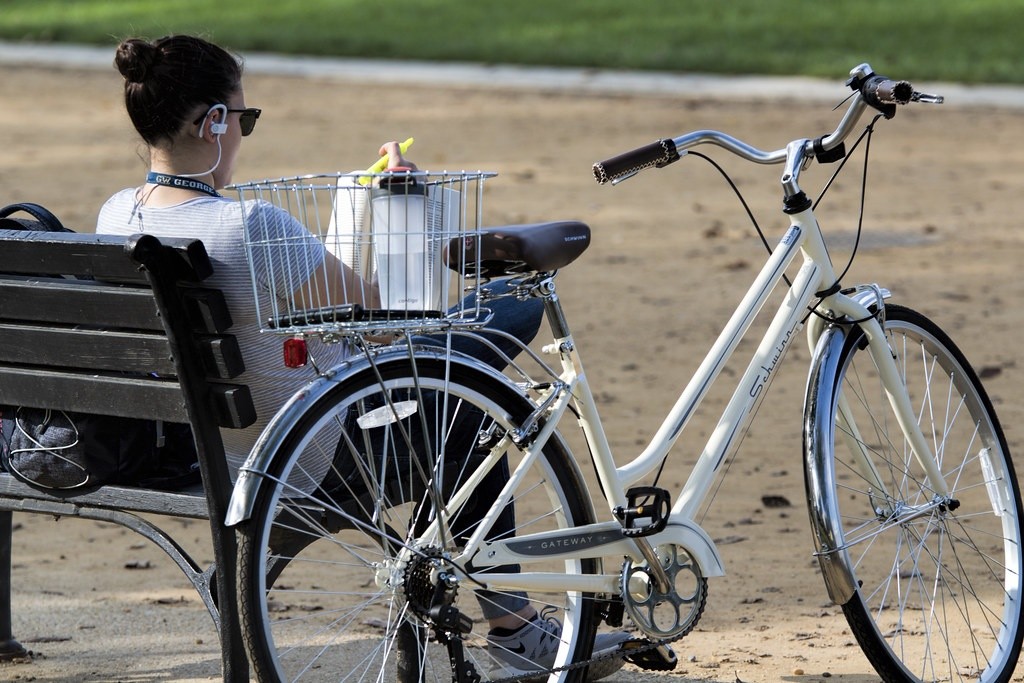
[325,170,459,313]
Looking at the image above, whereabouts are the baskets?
[225,170,501,335]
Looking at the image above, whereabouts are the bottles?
[371,166,429,312]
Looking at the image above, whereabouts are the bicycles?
[223,62,1024,682]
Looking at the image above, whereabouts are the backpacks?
[0,201,195,494]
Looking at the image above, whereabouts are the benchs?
[0,227,436,683]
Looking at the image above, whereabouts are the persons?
[96,35,635,683]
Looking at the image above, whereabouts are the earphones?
[210,122,228,134]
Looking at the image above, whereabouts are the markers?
[358,137,416,187]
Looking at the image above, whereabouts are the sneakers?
[485,604,631,683]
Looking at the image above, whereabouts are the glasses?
[192,101,261,137]
